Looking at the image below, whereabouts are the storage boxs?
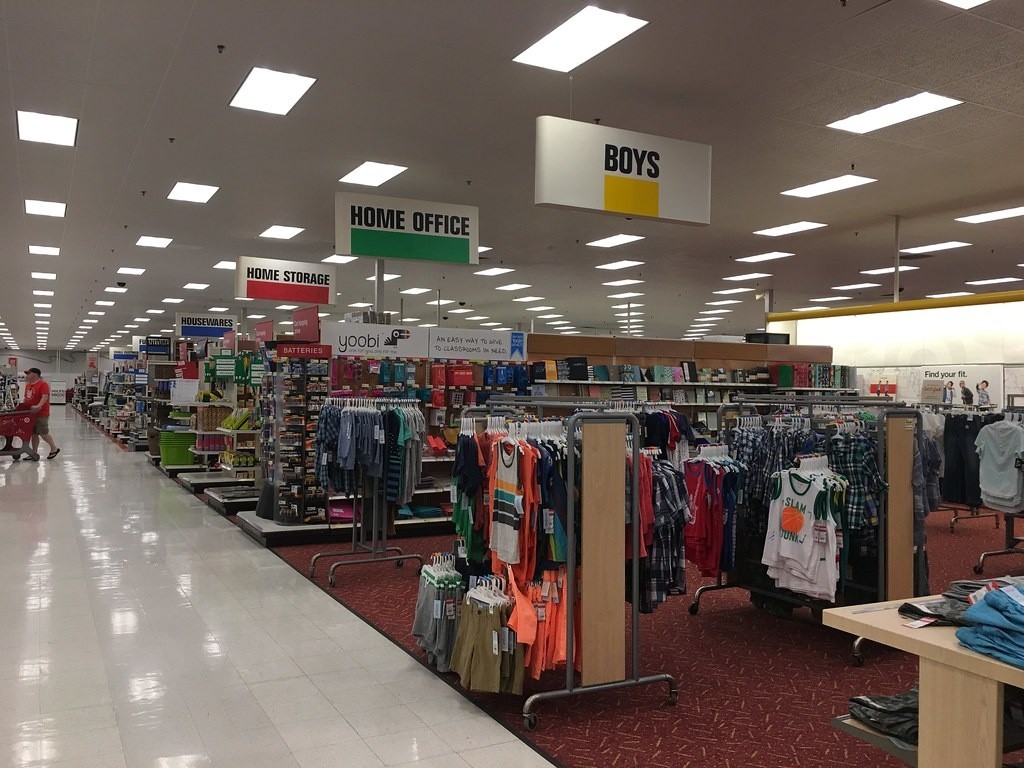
[167,378,199,403]
[158,432,197,467]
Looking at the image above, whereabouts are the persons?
[943,381,955,404]
[876,380,882,395]
[23,368,60,460]
[884,381,889,397]
[976,381,991,406]
[960,381,974,404]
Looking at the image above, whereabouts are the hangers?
[459,418,663,488]
[421,552,517,615]
[315,395,426,442]
[680,404,869,491]
[605,400,676,418]
[913,403,1024,427]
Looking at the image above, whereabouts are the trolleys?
[0,403,41,462]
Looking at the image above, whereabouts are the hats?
[24,368,41,375]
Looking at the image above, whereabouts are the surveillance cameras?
[459,302,466,306]
[899,288,904,292]
[443,317,448,321]
[117,282,126,287]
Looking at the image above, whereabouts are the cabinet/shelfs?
[201,424,276,511]
[144,392,178,467]
[535,379,778,405]
[71,371,146,451]
[180,397,236,486]
[822,593,1024,768]
[396,449,459,529]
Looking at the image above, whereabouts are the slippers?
[23,454,39,460]
[47,448,60,459]
